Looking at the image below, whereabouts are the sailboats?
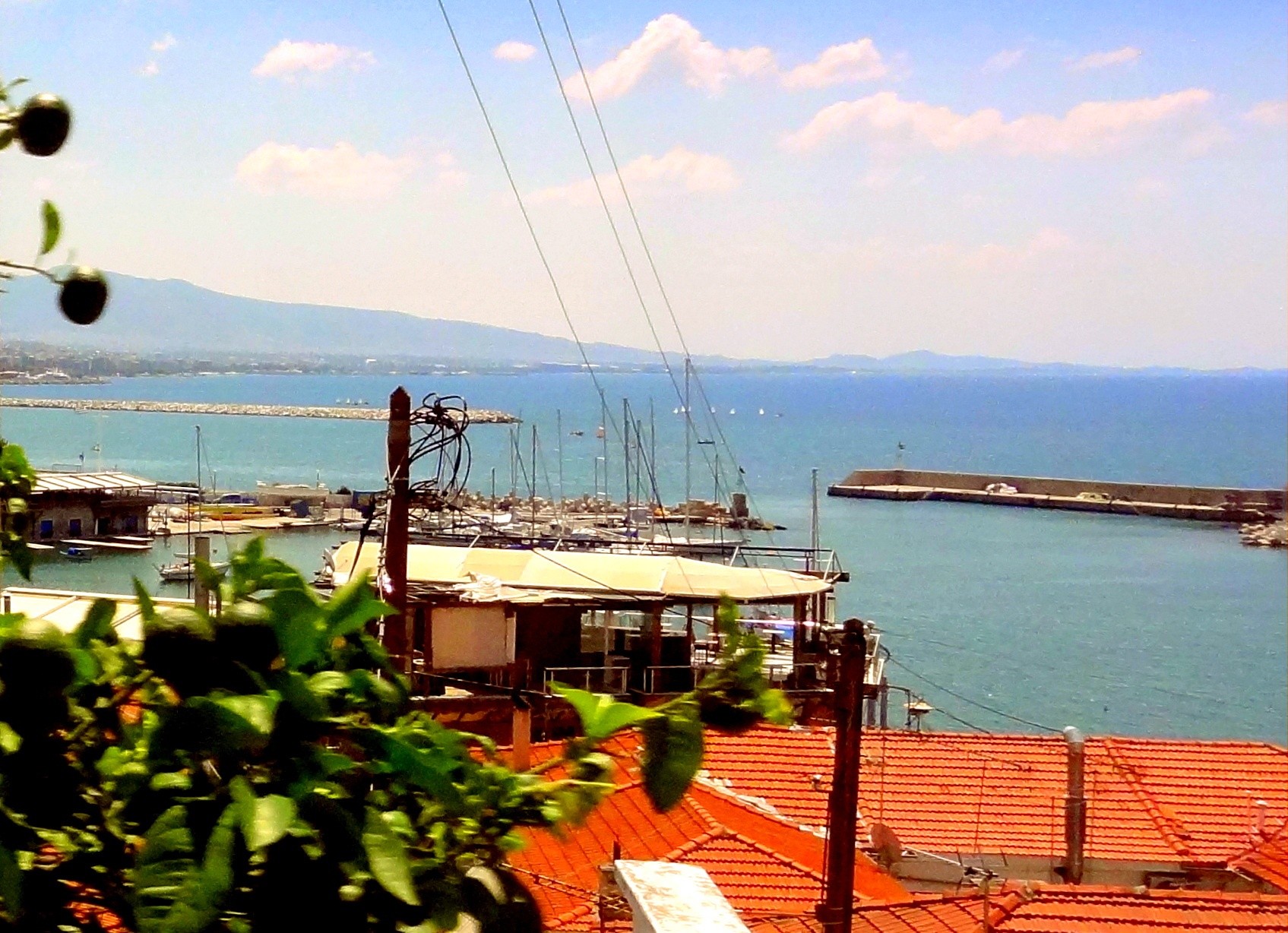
[153,423,233,582]
[328,357,934,755]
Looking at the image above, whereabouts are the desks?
[695,639,719,655]
[763,629,784,654]
